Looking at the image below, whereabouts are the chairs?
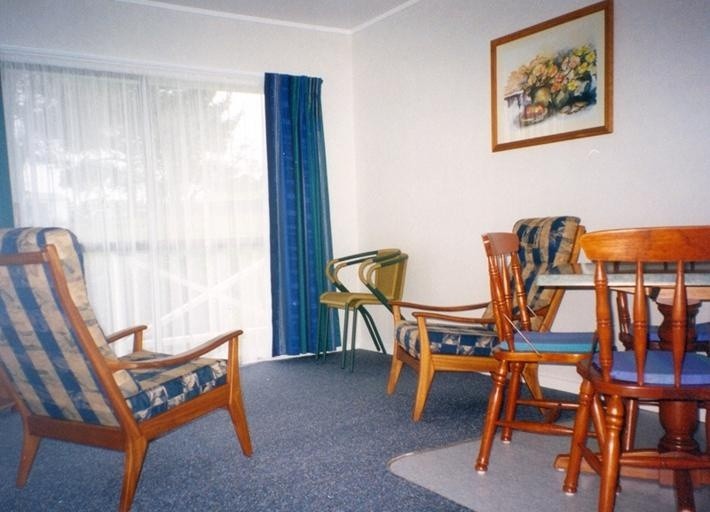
[0,221,255,511]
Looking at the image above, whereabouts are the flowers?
[514,40,597,110]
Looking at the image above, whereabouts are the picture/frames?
[488,0,614,153]
